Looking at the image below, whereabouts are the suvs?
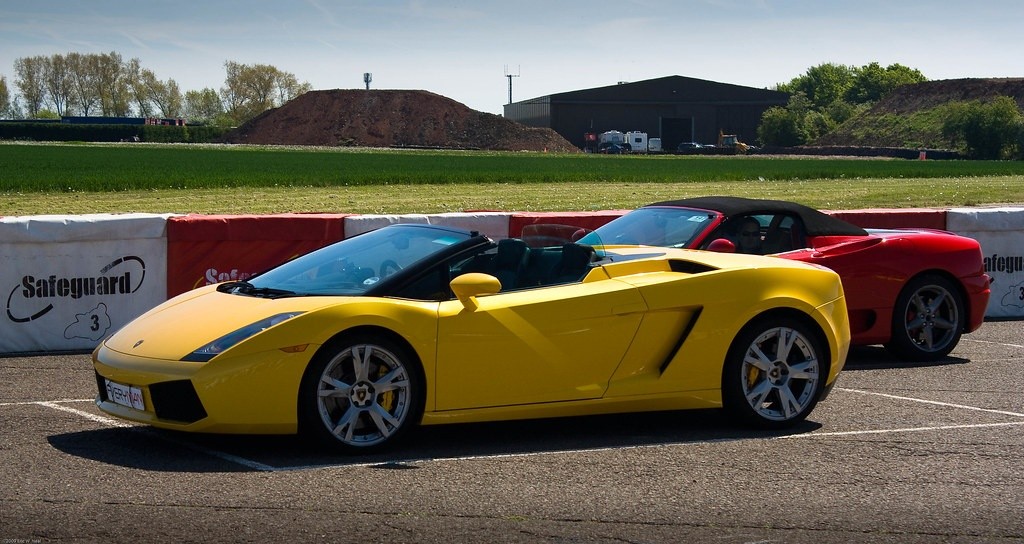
[678,143,697,153]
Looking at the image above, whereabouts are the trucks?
[649,138,661,154]
[600,130,623,154]
[691,127,749,154]
[623,130,647,154]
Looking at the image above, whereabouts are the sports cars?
[573,194,992,362]
[89,223,851,459]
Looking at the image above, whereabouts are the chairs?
[547,243,591,285]
[488,237,529,288]
[765,216,805,252]
